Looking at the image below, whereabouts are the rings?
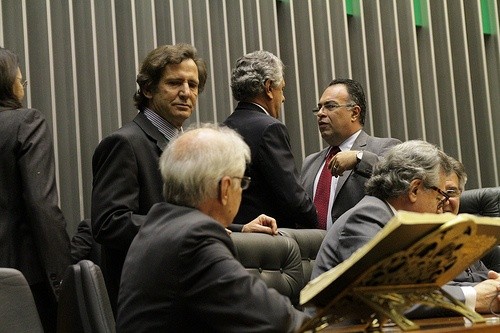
[333,165,338,170]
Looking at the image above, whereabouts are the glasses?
[429,186,449,209]
[218,175,251,190]
[445,189,461,199]
[311,100,354,116]
[16,77,28,89]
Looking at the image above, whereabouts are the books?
[299,209,500,310]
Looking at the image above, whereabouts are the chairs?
[229,232,306,313]
[277,228,327,286]
[457,186,500,274]
[55,259,116,333]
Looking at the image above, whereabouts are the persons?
[0,46,74,331]
[114,124,314,332]
[91,42,280,321]
[300,77,406,231]
[217,49,318,232]
[300,138,499,317]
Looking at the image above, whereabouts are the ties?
[314,146,341,230]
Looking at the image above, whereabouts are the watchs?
[356,149,365,165]
